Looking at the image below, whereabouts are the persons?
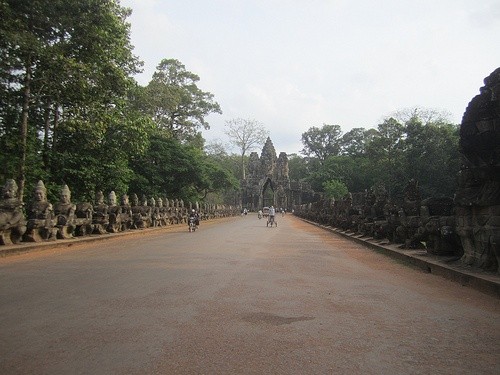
[51,183,76,240]
[0,178,28,245]
[23,179,56,242]
[75,201,94,235]
[292,67,500,273]
[258,209,263,218]
[89,190,109,235]
[269,206,276,221]
[106,191,241,232]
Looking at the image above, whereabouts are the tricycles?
[266,213,278,228]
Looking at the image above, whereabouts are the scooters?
[258,207,275,220]
[281,210,286,217]
[186,212,199,233]
